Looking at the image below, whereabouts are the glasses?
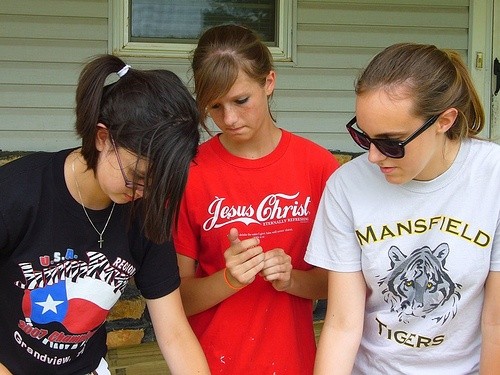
[111,135,146,191]
[345,114,439,159]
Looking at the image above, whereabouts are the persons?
[303,42,500,375]
[0,54,211,375]
[169,25,341,375]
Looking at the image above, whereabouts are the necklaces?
[72,160,116,248]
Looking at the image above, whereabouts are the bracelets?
[224,268,247,290]
